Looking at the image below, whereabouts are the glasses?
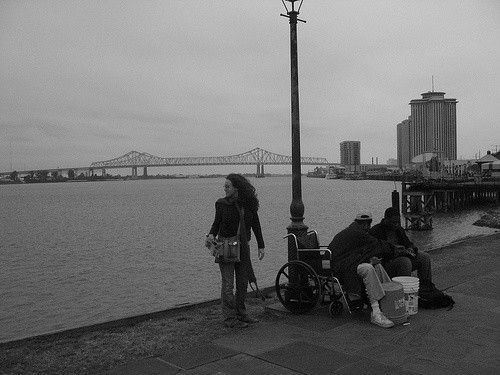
[224,185,235,188]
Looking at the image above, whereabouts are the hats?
[356,211,372,220]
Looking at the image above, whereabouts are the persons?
[204,172,266,329]
[324,211,406,329]
[368,206,455,310]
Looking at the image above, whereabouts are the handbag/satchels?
[340,264,362,303]
[215,235,240,263]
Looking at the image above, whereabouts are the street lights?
[280,0,310,301]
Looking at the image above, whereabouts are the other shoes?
[241,314,259,322]
[224,320,249,328]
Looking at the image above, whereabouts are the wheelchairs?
[275,228,365,319]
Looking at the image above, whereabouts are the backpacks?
[420,278,454,309]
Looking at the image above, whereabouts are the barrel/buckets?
[392,276,420,316]
[379,282,408,324]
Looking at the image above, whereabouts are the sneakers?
[371,311,394,328]
